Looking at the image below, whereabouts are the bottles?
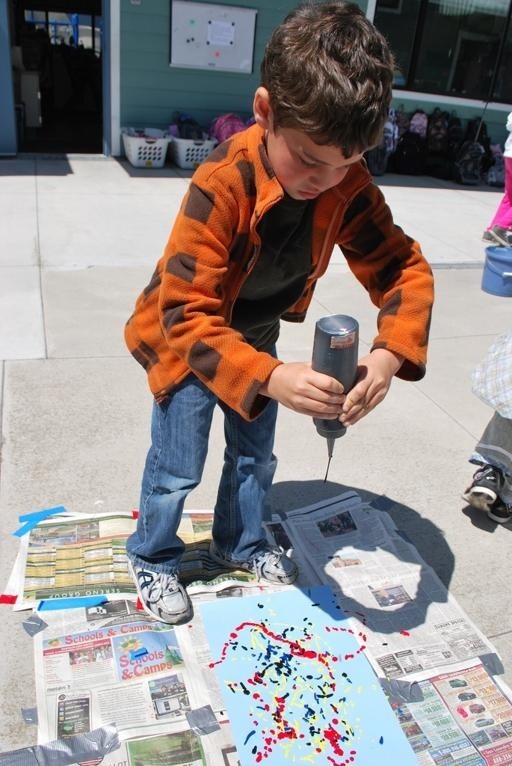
[311,314,359,459]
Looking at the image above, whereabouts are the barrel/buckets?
[481,246,512,298]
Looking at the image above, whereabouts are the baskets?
[122,127,171,168]
[170,137,219,170]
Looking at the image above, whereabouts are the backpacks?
[210,113,256,142]
[168,112,203,139]
[364,104,505,187]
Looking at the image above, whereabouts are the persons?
[124,0,434,625]
[460,113,512,523]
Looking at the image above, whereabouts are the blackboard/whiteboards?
[169,1,257,74]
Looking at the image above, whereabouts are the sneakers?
[483,225,512,245]
[209,538,299,584]
[128,559,190,624]
[463,464,512,524]
